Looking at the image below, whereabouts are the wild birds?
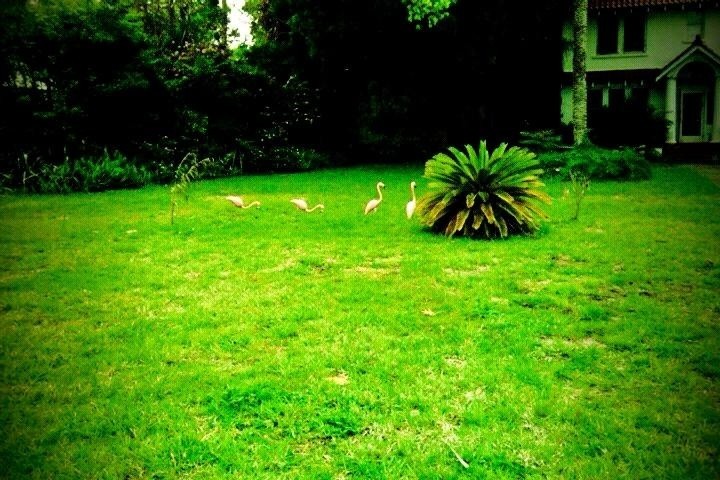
[226,195,261,210]
[290,198,324,214]
[406,181,416,219]
[365,182,385,216]
